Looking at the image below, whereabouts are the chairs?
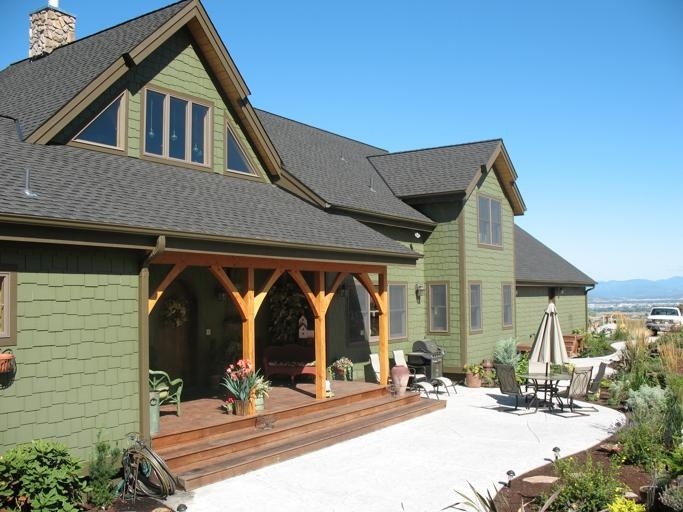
[393,350,427,393]
[525,359,550,403]
[369,353,416,391]
[149,368,183,417]
[493,364,539,412]
[549,366,593,413]
[587,362,607,394]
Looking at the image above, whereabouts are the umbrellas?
[525,299,568,408]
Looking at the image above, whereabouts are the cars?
[645,306,683,336]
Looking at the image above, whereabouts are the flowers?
[221,398,237,411]
[220,359,261,400]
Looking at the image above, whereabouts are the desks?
[517,373,571,413]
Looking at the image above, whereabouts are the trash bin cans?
[149,391,160,434]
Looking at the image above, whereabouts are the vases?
[226,410,233,415]
[390,364,409,396]
[235,398,255,416]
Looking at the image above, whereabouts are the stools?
[430,377,457,395]
[413,382,440,400]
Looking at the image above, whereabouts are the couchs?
[263,347,316,387]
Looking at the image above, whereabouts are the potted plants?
[255,375,273,410]
